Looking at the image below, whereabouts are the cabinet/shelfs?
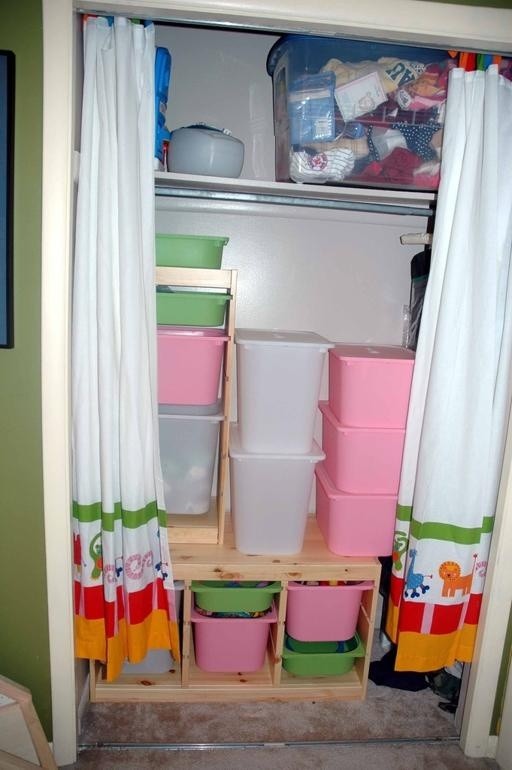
[85,513,383,704]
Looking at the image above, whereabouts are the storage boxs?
[228,327,333,555]
[120,581,375,676]
[314,343,416,557]
[266,36,459,192]
[152,234,233,557]
